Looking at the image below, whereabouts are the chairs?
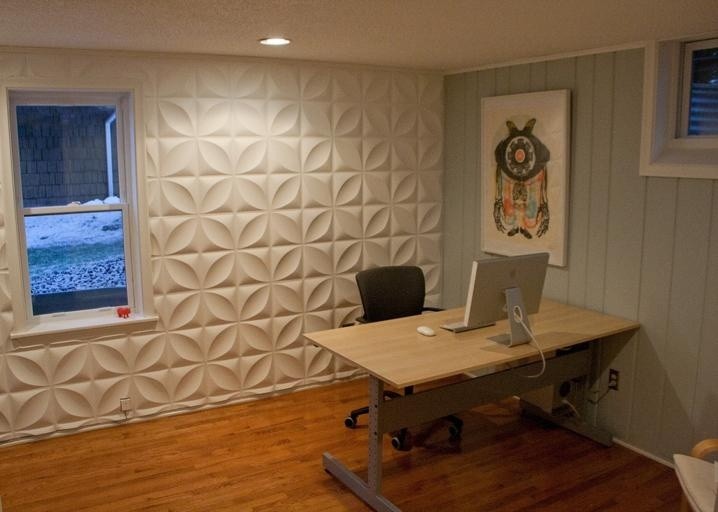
[343,264,464,448]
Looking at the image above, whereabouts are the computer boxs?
[520,379,578,418]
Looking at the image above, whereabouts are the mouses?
[417,325,435,337]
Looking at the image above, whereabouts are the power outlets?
[608,369,619,391]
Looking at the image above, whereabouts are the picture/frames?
[479,88,572,267]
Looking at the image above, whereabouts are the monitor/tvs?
[464,252,549,348]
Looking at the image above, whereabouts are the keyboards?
[440,322,496,333]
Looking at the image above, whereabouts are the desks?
[300,295,640,512]
